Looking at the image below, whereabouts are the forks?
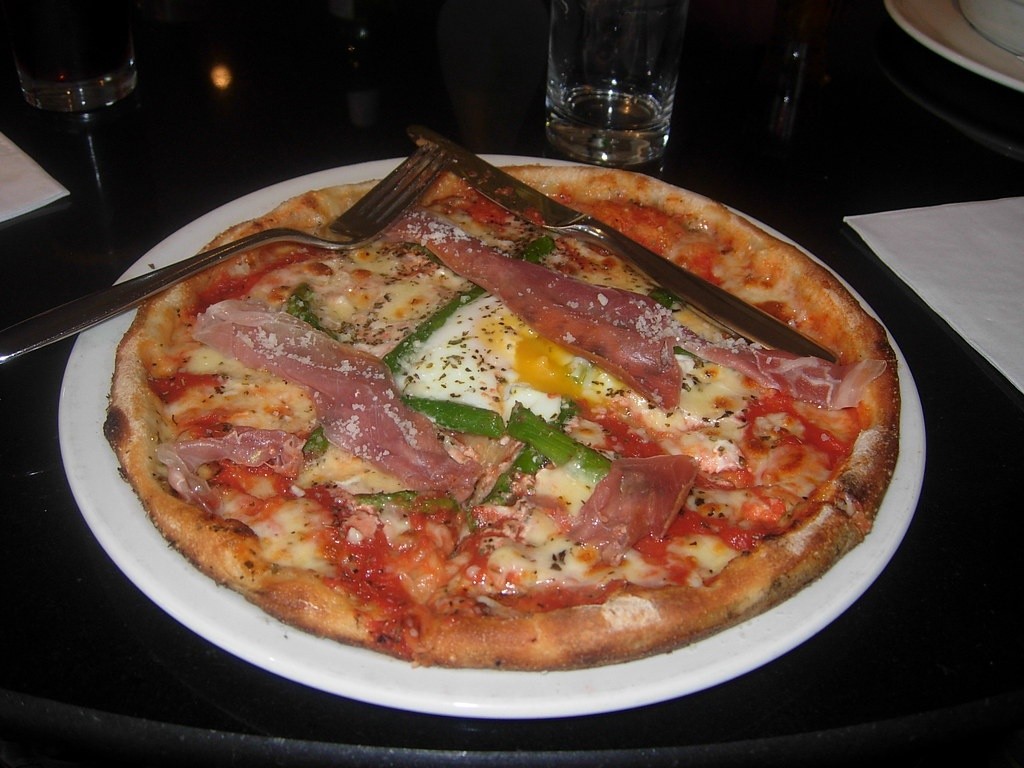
[0,140,450,364]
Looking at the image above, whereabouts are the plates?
[57,152,926,717]
[884,0,1024,92]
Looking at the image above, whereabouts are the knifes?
[411,129,838,365]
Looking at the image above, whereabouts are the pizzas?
[104,163,901,669]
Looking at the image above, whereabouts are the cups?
[0,0,136,113]
[545,0,686,166]
